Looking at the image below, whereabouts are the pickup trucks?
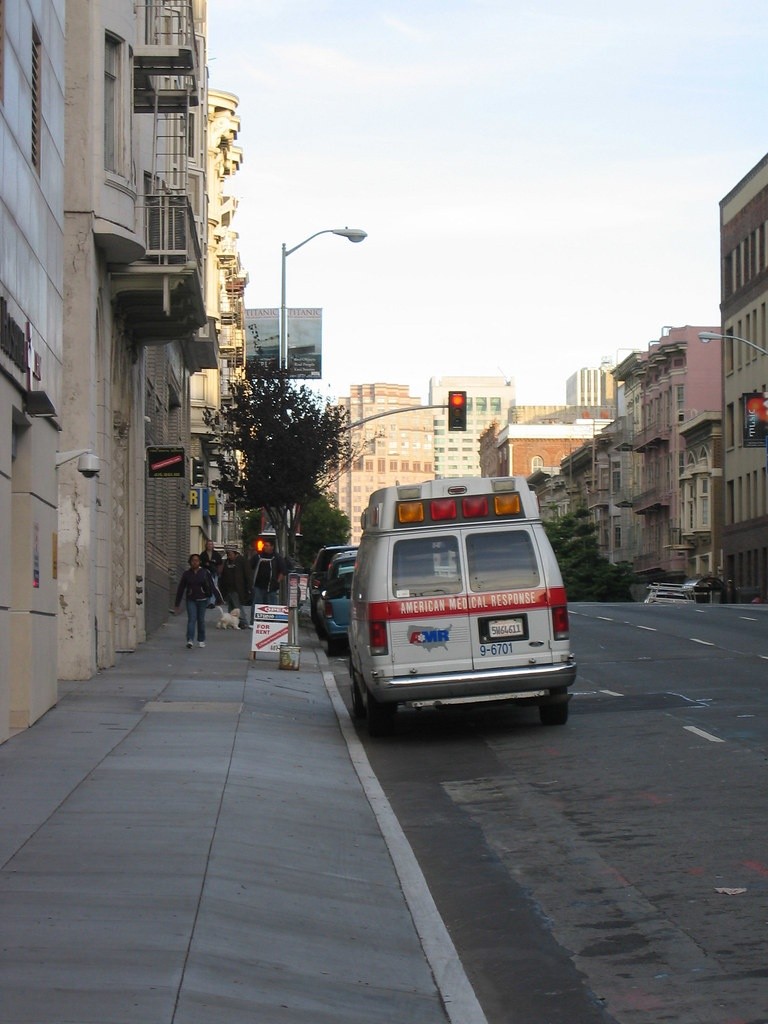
[644,583,696,605]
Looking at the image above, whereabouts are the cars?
[311,550,358,655]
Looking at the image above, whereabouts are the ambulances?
[318,474,577,738]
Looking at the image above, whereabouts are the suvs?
[302,545,358,625]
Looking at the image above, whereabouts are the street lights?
[279,228,367,600]
[544,478,553,498]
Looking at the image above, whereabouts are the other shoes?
[210,604,215,609]
[198,642,205,648]
[187,641,193,649]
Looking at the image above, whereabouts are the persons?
[222,544,250,628]
[198,541,223,608]
[174,554,215,648]
[248,539,288,628]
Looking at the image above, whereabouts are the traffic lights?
[448,391,466,430]
[256,539,264,553]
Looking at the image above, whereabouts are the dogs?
[215,605,241,629]
